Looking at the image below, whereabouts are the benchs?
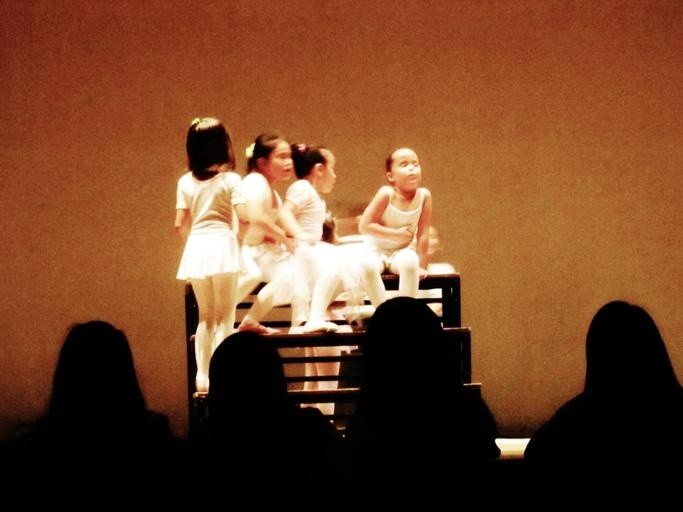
[183,270,484,435]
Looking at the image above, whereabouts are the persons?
[413,223,458,327]
[178,330,360,511]
[297,213,358,437]
[1,319,184,512]
[519,297,683,510]
[277,140,363,333]
[342,294,503,512]
[234,131,315,336]
[172,116,252,395]
[357,148,434,308]
